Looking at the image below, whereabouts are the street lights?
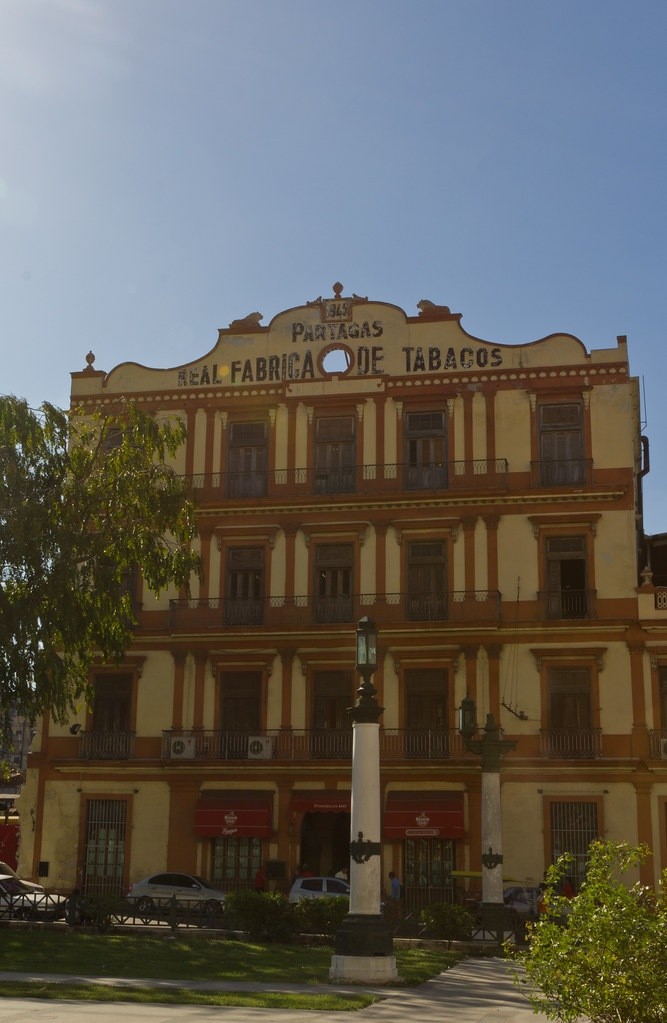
[329,616,398,980]
[459,696,523,943]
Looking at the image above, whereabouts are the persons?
[255,865,268,891]
[296,863,314,877]
[388,872,400,898]
[538,872,559,895]
[335,867,347,879]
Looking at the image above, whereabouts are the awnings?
[289,791,351,813]
[383,790,464,839]
[195,799,272,838]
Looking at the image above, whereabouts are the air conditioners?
[660,739,667,759]
[170,736,196,759]
[248,736,276,759]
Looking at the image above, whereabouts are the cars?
[288,877,350,905]
[504,886,540,914]
[0,861,44,894]
[0,874,69,922]
[125,872,229,918]
